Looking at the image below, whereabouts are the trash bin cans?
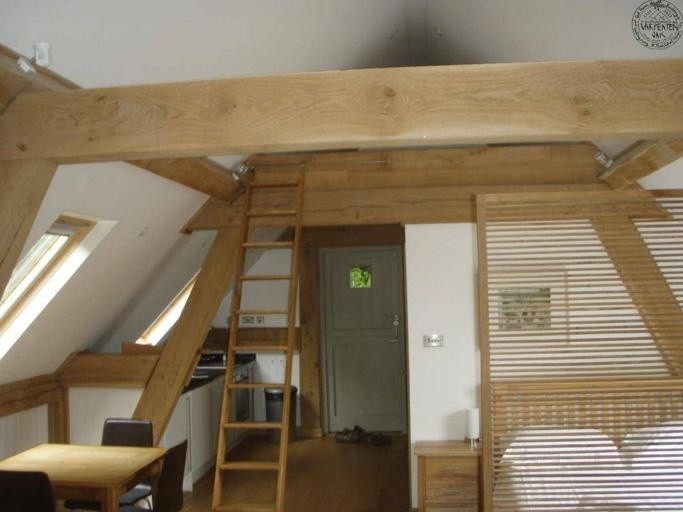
[264,383,297,443]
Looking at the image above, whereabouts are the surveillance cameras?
[19,58,35,78]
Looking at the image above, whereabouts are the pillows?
[625,419,683,471]
[499,426,622,471]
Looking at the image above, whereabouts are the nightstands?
[417,440,481,512]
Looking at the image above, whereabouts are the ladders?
[210,166,305,512]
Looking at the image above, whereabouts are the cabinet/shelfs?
[67,352,254,492]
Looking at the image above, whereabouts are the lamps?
[465,407,481,448]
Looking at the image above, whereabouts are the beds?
[489,379,683,512]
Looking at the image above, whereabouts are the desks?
[0,443,169,512]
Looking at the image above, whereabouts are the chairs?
[65,420,153,512]
[119,440,187,512]
[0,469,56,512]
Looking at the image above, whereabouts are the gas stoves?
[198,353,257,367]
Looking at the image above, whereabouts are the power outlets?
[423,333,442,348]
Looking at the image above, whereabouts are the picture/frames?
[478,268,568,344]
